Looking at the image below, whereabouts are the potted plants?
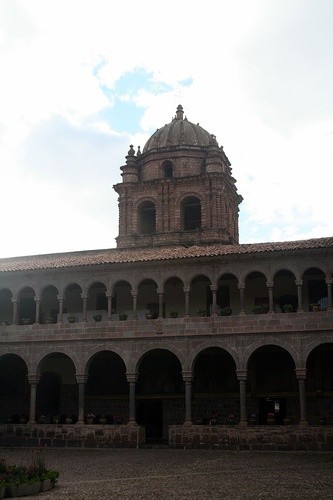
[1,300,332,497]
